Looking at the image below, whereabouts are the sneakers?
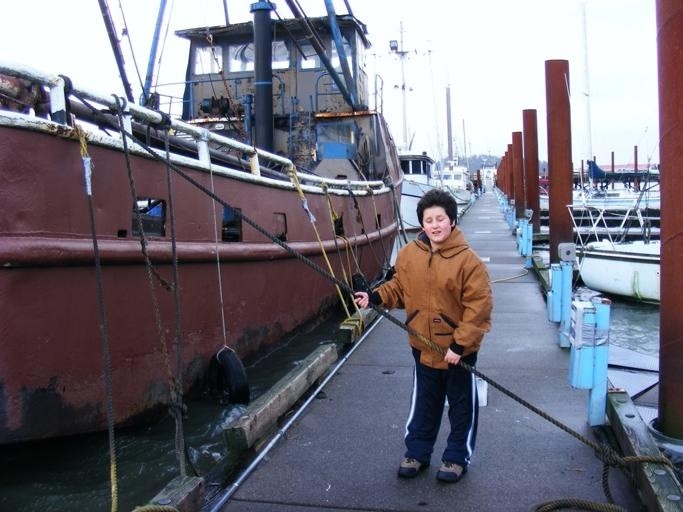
[397,456,423,478]
[436,459,464,483]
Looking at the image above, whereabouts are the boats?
[540,190,661,303]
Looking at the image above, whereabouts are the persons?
[351,187,493,485]
